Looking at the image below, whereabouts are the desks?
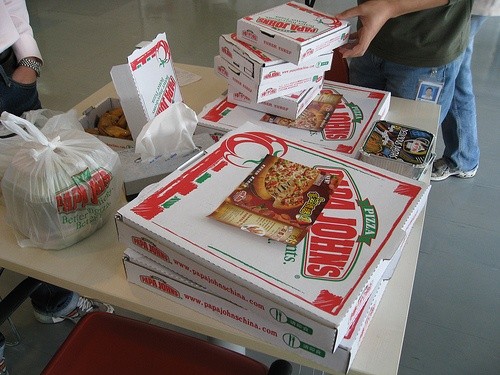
[0,63,442,375]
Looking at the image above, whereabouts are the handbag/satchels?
[0,108,122,250]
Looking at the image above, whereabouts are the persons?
[430,0,500,181]
[0,0,115,375]
[421,87,433,99]
[335,0,474,126]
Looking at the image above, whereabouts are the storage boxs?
[79,0,431,375]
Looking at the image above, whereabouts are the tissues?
[115,102,217,202]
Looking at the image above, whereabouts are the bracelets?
[19,58,41,77]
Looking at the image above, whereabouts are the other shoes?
[431,158,479,181]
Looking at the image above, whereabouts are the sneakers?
[0,358,9,375]
[33,295,116,324]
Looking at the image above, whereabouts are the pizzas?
[253,155,320,209]
[272,109,318,130]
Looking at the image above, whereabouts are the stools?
[40,312,269,375]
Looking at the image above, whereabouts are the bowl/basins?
[1,129,121,250]
